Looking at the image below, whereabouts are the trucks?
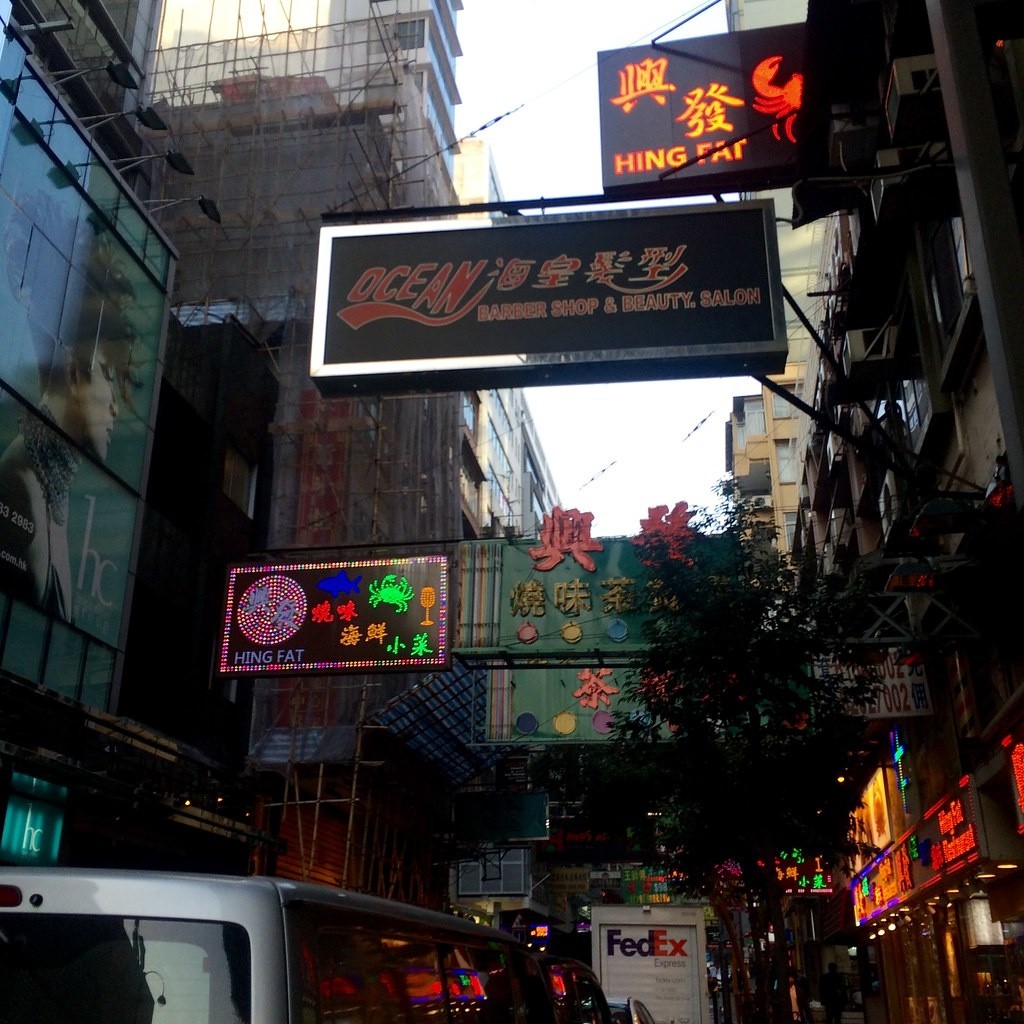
[587,900,750,1024]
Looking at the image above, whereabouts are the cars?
[574,991,656,1024]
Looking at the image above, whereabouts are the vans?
[530,949,613,1024]
[0,863,557,1024]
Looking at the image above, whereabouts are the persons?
[819,963,848,1024]
[749,963,815,1024]
